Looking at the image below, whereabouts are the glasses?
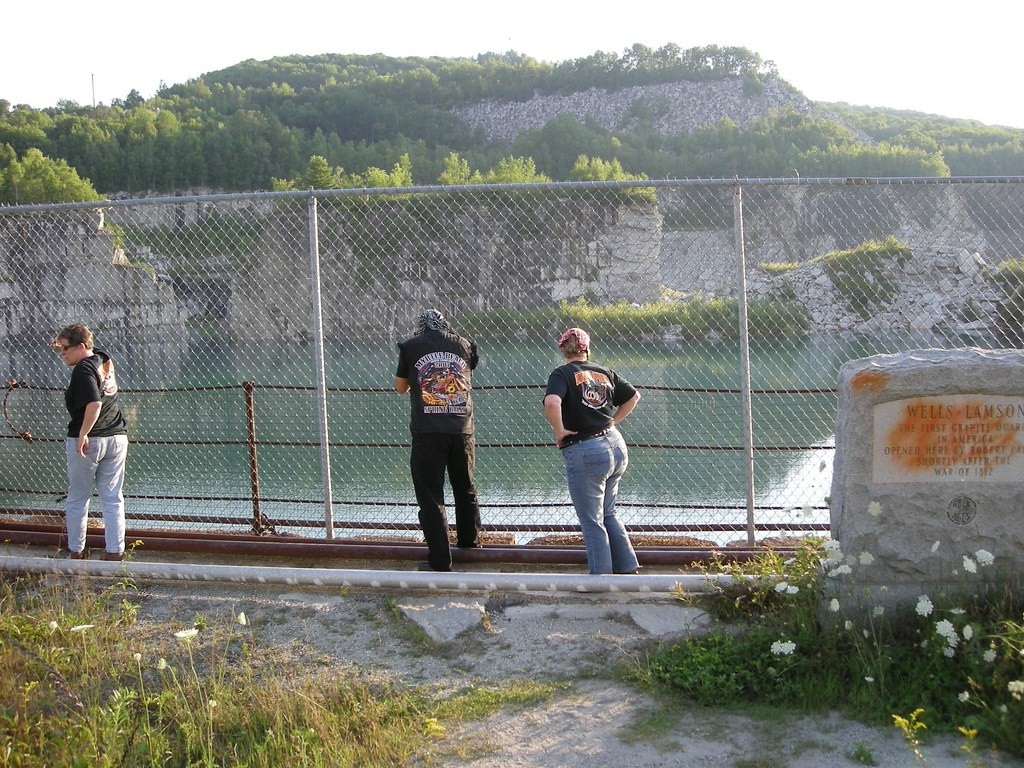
[60,343,79,351]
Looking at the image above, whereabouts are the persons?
[396,309,481,571]
[542,328,641,573]
[57,324,129,561]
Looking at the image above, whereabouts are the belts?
[559,426,616,450]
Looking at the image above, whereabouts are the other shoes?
[100,549,122,561]
[418,563,450,571]
[71,548,90,559]
